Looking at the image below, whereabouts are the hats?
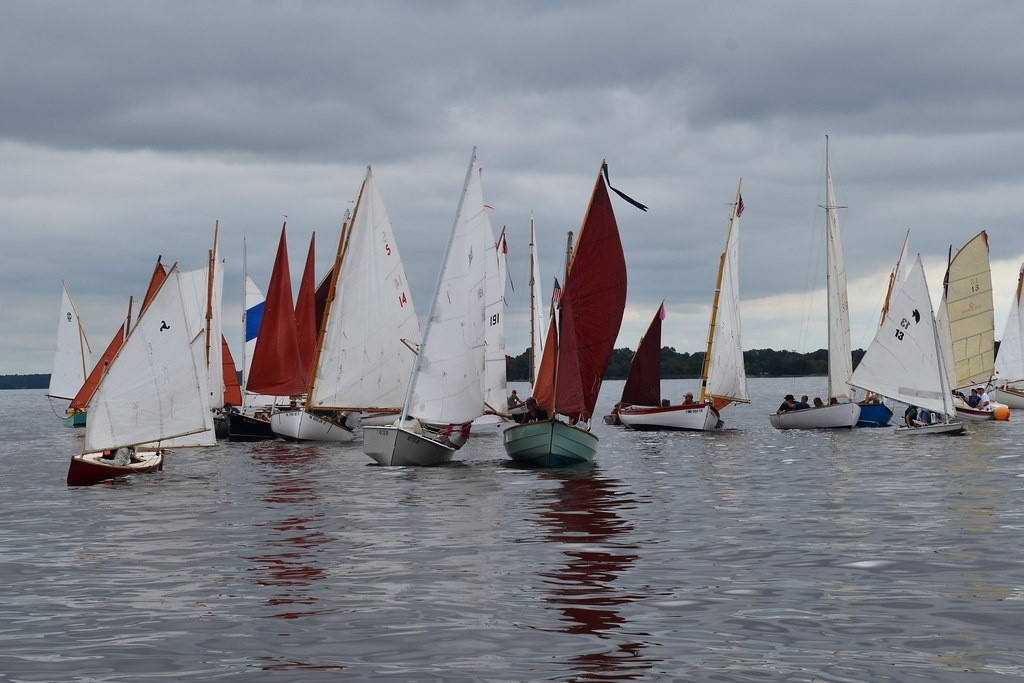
[784,395,795,400]
[977,384,984,389]
[512,390,516,394]
[683,393,693,398]
[526,398,537,404]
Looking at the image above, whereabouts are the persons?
[338,410,362,429]
[433,419,472,450]
[813,397,829,408]
[223,403,241,414]
[784,395,810,410]
[968,388,981,409]
[951,389,966,402]
[572,417,591,431]
[212,407,223,418]
[918,408,942,424]
[778,393,800,411]
[507,389,525,410]
[609,402,621,414]
[255,409,269,421]
[680,393,695,405]
[289,400,300,411]
[63,407,85,415]
[976,384,990,411]
[904,404,918,428]
[483,396,549,425]
[96,443,135,467]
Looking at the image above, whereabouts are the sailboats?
[990,261,1024,410]
[505,209,592,431]
[606,297,668,426]
[857,227,912,427]
[931,228,1011,422]
[768,134,862,430]
[45,144,507,486]
[844,225,968,438]
[501,160,649,470]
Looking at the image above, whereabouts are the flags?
[659,303,666,320]
[736,193,745,218]
[496,233,508,254]
[552,279,561,302]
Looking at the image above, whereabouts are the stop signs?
[617,176,752,431]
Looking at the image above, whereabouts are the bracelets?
[494,411,496,415]
